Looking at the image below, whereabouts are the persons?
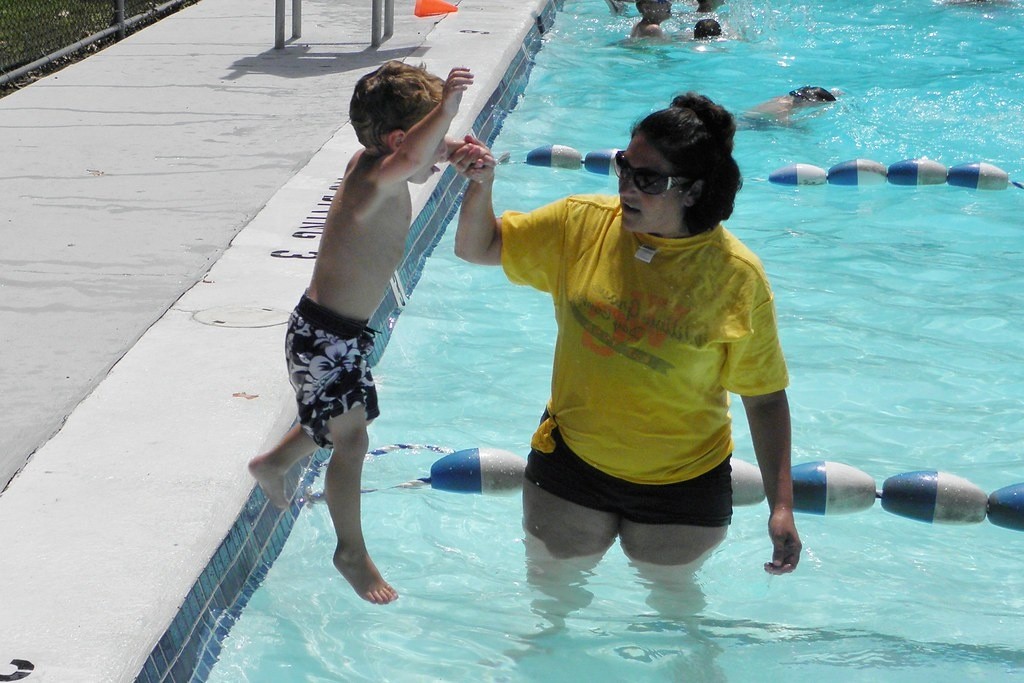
[450,92,802,576]
[248,59,489,604]
[606,0,735,43]
[789,86,836,107]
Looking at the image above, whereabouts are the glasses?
[648,0,668,4]
[614,151,698,195]
